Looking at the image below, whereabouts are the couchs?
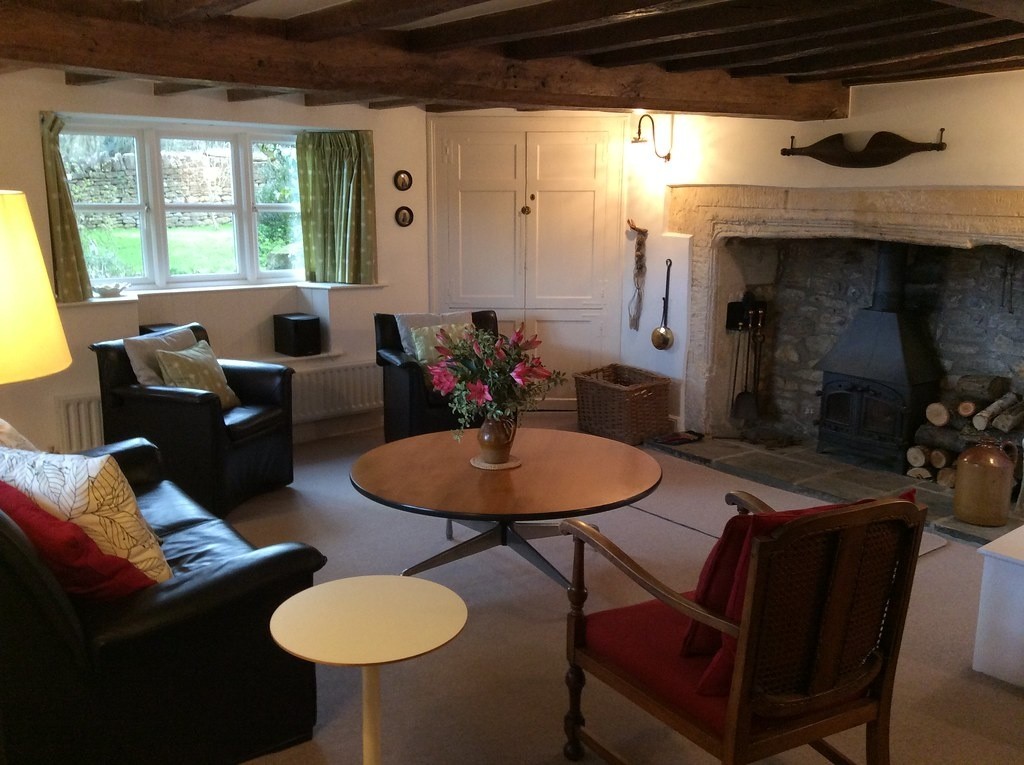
[0,437,328,765]
[88,322,297,515]
[550,490,929,765]
[374,310,517,443]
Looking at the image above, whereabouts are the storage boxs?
[273,313,321,357]
[139,322,176,336]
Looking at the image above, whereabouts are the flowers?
[428,321,569,443]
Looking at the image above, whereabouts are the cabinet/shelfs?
[972,524,1024,686]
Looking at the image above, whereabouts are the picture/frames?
[396,206,414,227]
[394,170,413,191]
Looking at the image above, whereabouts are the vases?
[477,416,516,463]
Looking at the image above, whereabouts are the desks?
[269,576,468,765]
[348,428,662,576]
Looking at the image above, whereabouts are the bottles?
[952,436,1018,528]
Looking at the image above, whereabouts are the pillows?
[122,329,242,414]
[394,311,474,394]
[0,447,175,604]
[678,486,917,693]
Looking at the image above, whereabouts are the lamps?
[630,112,674,164]
[0,190,72,385]
